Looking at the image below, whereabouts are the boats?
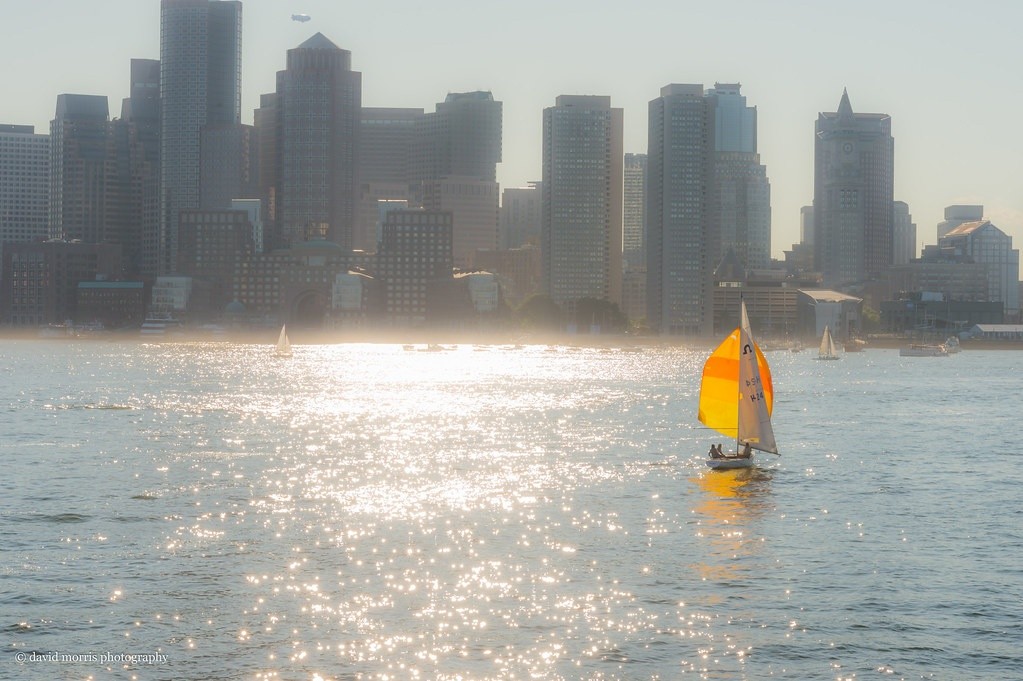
[899,342,949,358]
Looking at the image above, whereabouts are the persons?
[708,444,722,458]
[716,444,726,457]
[742,443,752,459]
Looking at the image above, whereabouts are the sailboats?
[267,324,294,359]
[814,323,842,361]
[695,294,783,470]
